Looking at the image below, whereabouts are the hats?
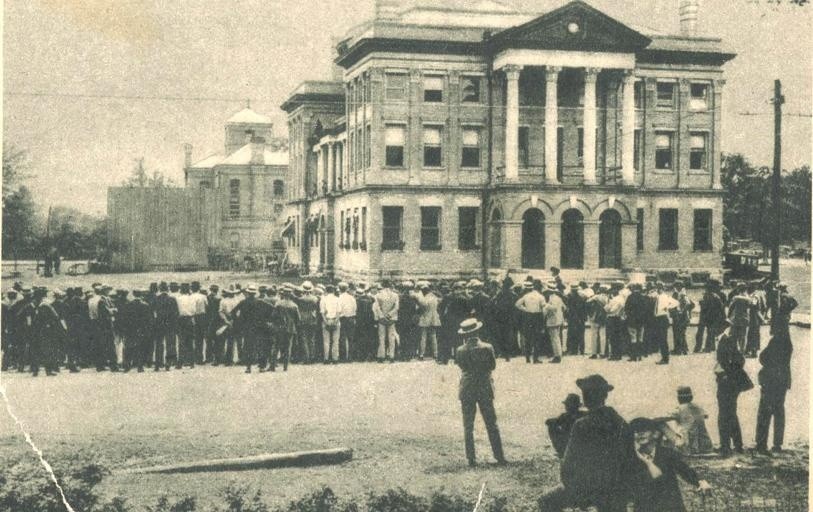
[562,393,582,407]
[576,373,614,392]
[456,316,483,336]
[676,385,694,399]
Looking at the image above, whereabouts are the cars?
[722,253,771,284]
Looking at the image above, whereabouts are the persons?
[622,418,712,511]
[2,246,798,378]
[755,299,804,455]
[709,307,750,454]
[453,316,508,468]
[535,374,630,512]
